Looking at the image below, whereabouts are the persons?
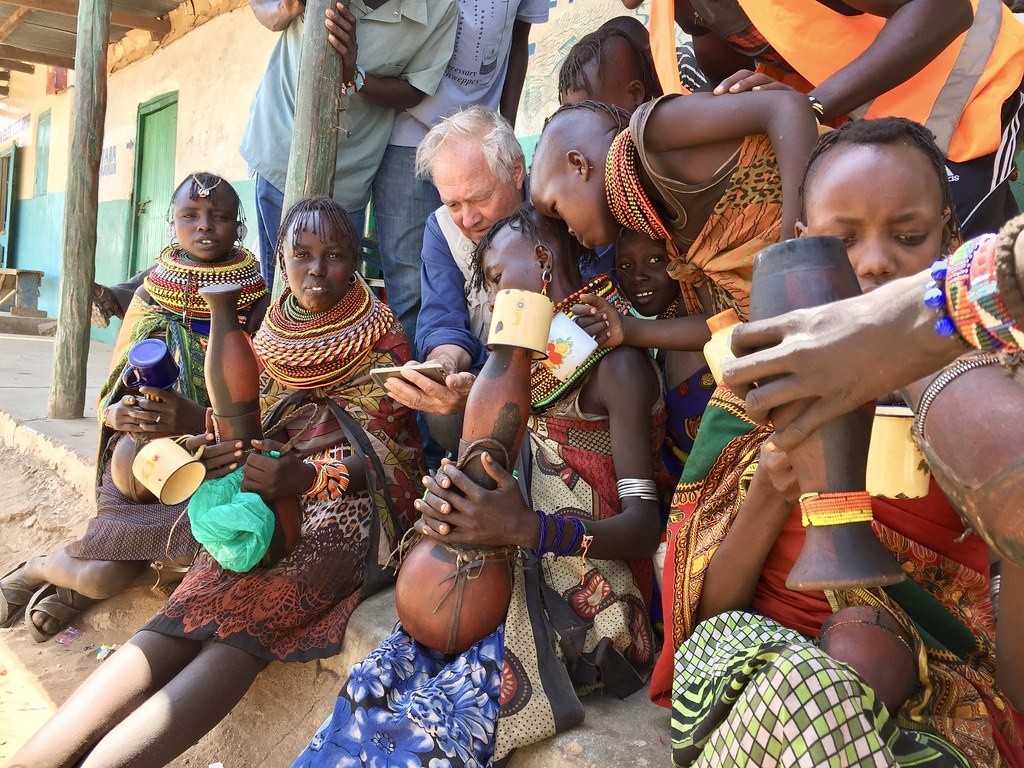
[292,206,660,768]
[373,0,550,476]
[718,210,1024,451]
[0,171,271,644]
[2,193,428,768]
[620,0,1024,243]
[646,113,1024,768]
[238,0,460,295]
[383,105,615,451]
[557,14,666,121]
[615,222,715,530]
[524,83,844,351]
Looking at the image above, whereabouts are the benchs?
[0,268,47,318]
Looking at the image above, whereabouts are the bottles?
[701,308,747,387]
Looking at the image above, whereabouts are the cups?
[481,289,554,360]
[122,338,180,389]
[865,405,931,499]
[537,310,599,384]
[132,435,207,506]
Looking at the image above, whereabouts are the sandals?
[91,281,125,329]
[0,555,97,642]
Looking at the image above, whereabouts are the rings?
[153,412,162,423]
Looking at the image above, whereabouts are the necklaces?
[248,273,392,389]
[142,240,268,319]
[603,124,671,240]
[528,273,631,408]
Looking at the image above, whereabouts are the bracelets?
[805,94,826,126]
[910,351,1003,437]
[530,508,591,558]
[918,212,1023,381]
[338,64,367,97]
